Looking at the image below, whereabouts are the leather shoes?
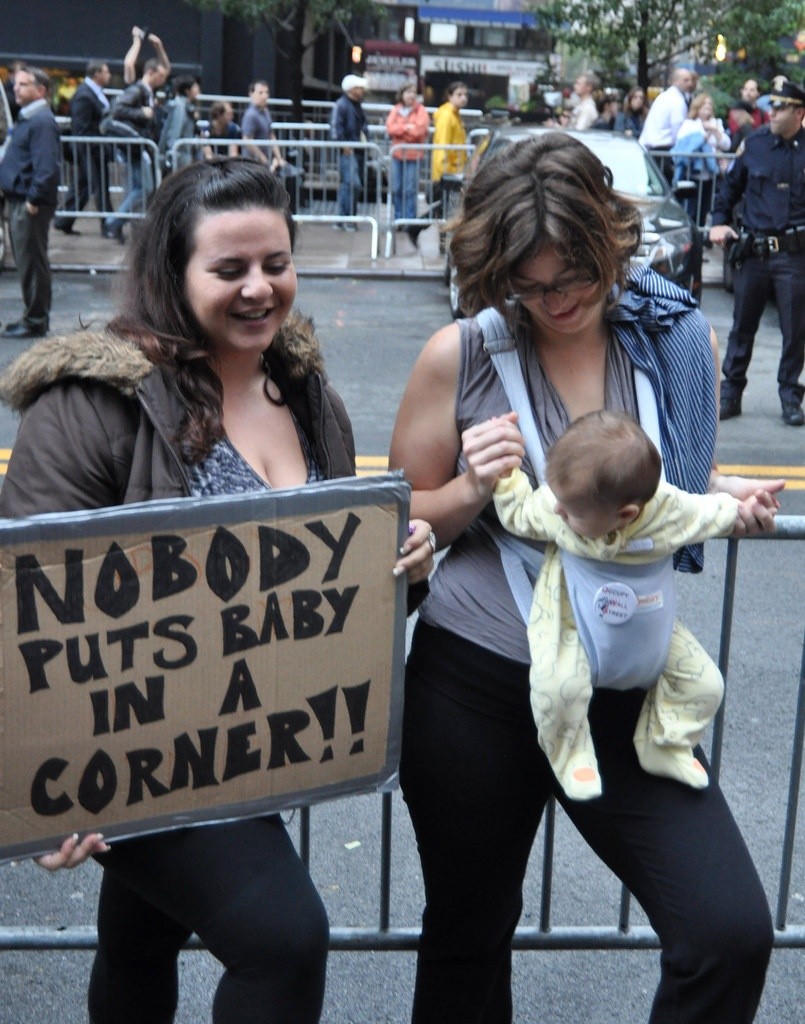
[782,403,805,425]
[719,397,743,419]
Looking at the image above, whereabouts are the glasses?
[506,266,603,302]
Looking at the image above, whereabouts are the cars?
[445,127,701,320]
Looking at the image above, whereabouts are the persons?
[638,67,805,258]
[708,79,804,426]
[480,409,782,804]
[542,70,649,139]
[0,65,62,337]
[389,125,796,1024]
[104,27,288,243]
[385,83,429,232]
[404,81,469,255]
[331,74,371,234]
[0,154,435,1024]
[51,58,117,238]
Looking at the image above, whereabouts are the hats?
[341,74,367,93]
[766,77,805,109]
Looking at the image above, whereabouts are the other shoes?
[335,223,360,234]
[55,223,80,235]
[1,316,46,339]
[105,222,125,245]
[405,226,420,251]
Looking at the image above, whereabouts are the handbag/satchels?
[99,87,151,154]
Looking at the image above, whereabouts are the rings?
[428,532,437,552]
